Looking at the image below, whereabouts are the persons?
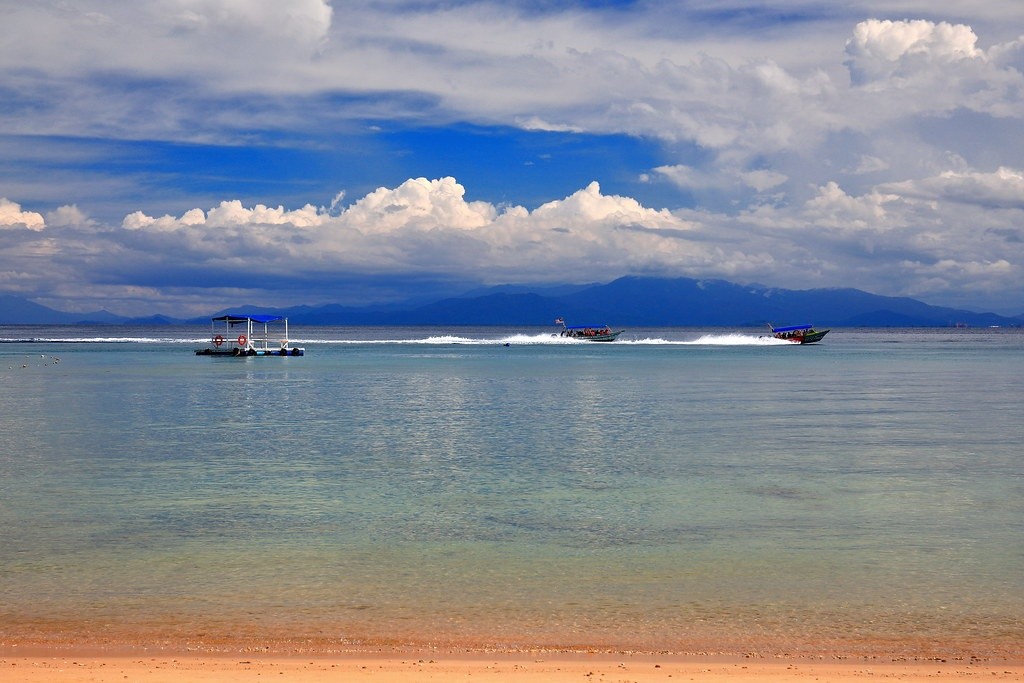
[566,327,609,337]
[775,329,813,338]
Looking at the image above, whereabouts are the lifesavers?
[215,335,222,345]
[238,334,245,345]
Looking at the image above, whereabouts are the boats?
[759,322,832,343]
[194,311,306,357]
[551,317,626,342]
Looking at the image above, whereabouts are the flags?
[555,317,563,324]
